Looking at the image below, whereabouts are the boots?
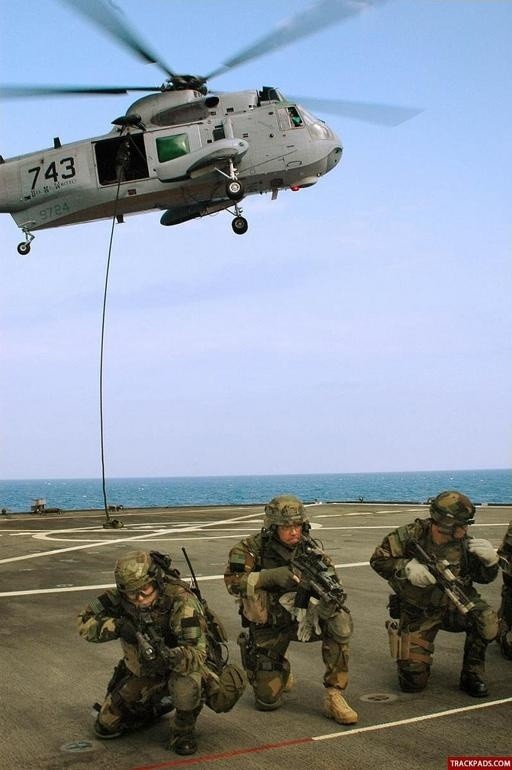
[172,709,198,756]
[459,666,488,698]
[320,687,358,724]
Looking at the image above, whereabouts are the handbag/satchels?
[209,662,249,713]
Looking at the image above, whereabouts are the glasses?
[123,581,157,601]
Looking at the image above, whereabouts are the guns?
[405,541,487,627]
[120,605,169,663]
[290,548,351,615]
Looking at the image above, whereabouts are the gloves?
[403,558,438,588]
[467,535,501,568]
[259,565,301,590]
[315,602,337,621]
[115,617,141,644]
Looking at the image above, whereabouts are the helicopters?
[0,2,423,254]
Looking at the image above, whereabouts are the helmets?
[428,490,475,535]
[114,552,166,606]
[263,496,311,533]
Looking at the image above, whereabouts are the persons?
[369,490,500,699]
[224,492,360,726]
[494,519,511,662]
[77,550,226,757]
